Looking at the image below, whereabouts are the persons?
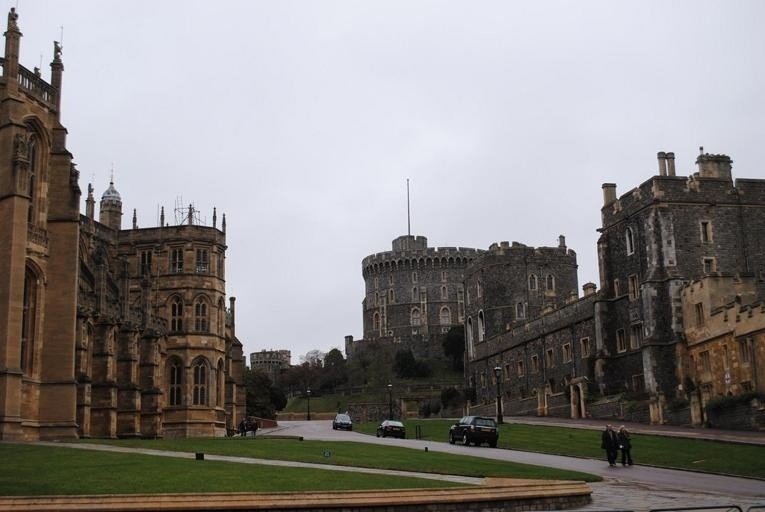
[616,425,634,466]
[250,418,258,439]
[600,423,619,466]
[239,418,248,437]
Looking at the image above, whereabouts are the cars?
[376,420,405,439]
[448,415,499,448]
[333,414,352,430]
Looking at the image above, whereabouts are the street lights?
[387,379,393,420]
[306,385,312,420]
[494,360,503,425]
